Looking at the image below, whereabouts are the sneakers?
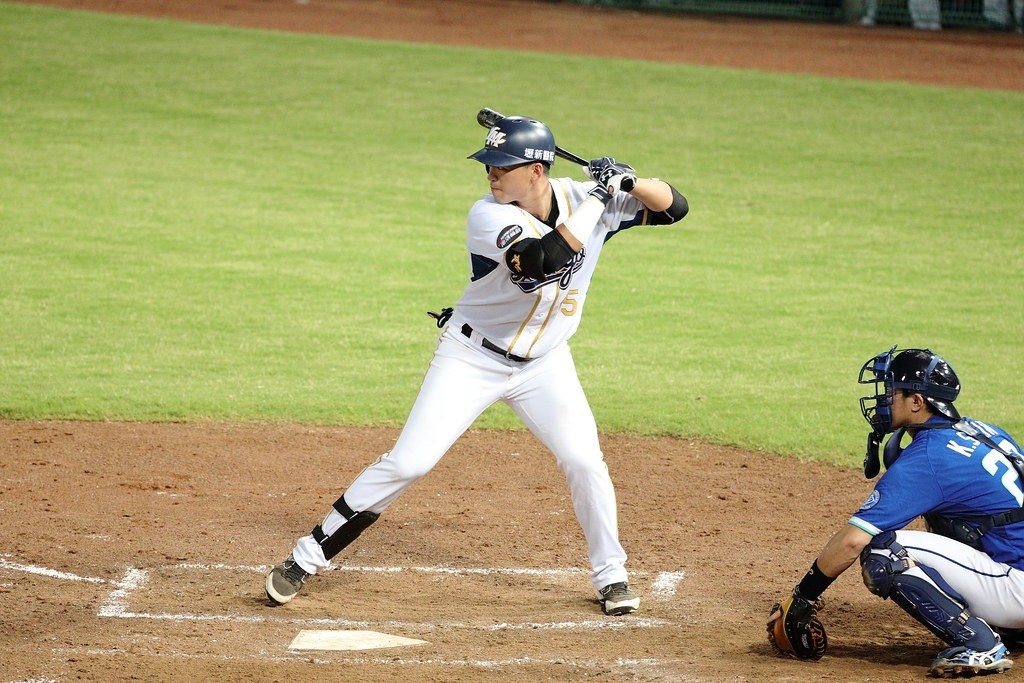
[599,582,639,616]
[267,553,312,607]
[929,640,1013,677]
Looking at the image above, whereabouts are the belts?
[446,312,534,363]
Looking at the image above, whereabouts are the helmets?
[889,349,961,420]
[467,116,556,166]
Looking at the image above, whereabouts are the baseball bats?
[476,105,638,194]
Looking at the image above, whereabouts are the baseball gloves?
[765,584,829,662]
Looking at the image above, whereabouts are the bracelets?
[798,557,837,601]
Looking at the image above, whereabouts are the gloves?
[588,162,636,208]
[583,157,637,193]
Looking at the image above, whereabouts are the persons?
[265,117,690,616]
[766,343,1024,676]
